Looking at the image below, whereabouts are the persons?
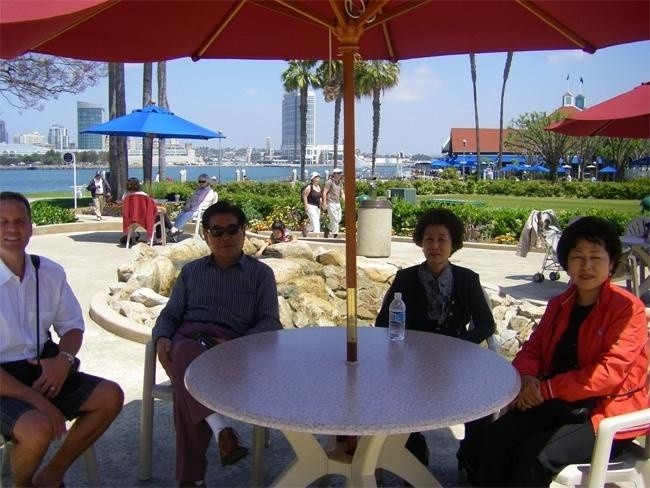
[123,176,184,245]
[302,167,346,240]
[256,219,297,256]
[171,173,216,240]
[455,215,650,487]
[150,199,282,488]
[88,170,112,221]
[0,191,124,488]
[371,209,495,345]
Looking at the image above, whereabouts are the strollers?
[528,206,568,284]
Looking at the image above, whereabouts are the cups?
[175,194,180,202]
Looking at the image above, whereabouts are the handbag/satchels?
[300,184,313,202]
[536,364,592,423]
[120,232,141,244]
[37,339,81,387]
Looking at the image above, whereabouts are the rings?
[50,385,56,389]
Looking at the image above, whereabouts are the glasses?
[207,224,239,237]
[199,181,205,184]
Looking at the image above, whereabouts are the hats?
[96,170,101,175]
[310,171,320,180]
[332,168,343,173]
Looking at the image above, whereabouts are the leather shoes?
[219,427,248,467]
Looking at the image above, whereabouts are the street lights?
[461,137,470,180]
[586,164,595,179]
[395,151,412,179]
[473,161,495,179]
[562,164,572,177]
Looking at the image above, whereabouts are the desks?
[184,327,521,488]
[620,235,650,297]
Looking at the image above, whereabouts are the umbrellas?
[541,80,650,139]
[430,153,617,182]
[81,101,226,171]
[0,0,650,363]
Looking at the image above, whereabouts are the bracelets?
[59,349,75,366]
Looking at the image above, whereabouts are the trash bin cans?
[356,199,393,257]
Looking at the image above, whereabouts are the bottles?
[388,293,406,342]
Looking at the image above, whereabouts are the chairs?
[499,405,650,488]
[0,435,101,488]
[468,316,499,425]
[123,195,166,248]
[611,256,639,298]
[179,192,218,236]
[625,218,650,292]
[138,342,271,488]
